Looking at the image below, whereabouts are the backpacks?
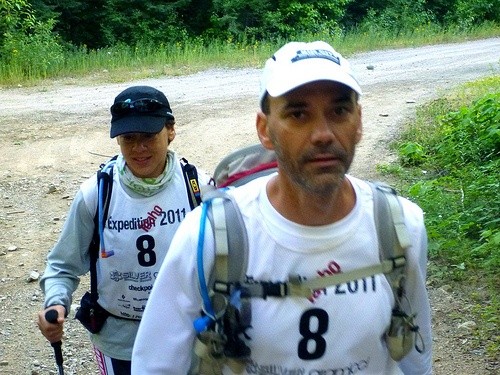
[193,143,412,375]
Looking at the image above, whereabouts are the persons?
[37,86,213,375]
[130,41,432,375]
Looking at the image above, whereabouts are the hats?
[109,85,175,138]
[259,40,363,112]
